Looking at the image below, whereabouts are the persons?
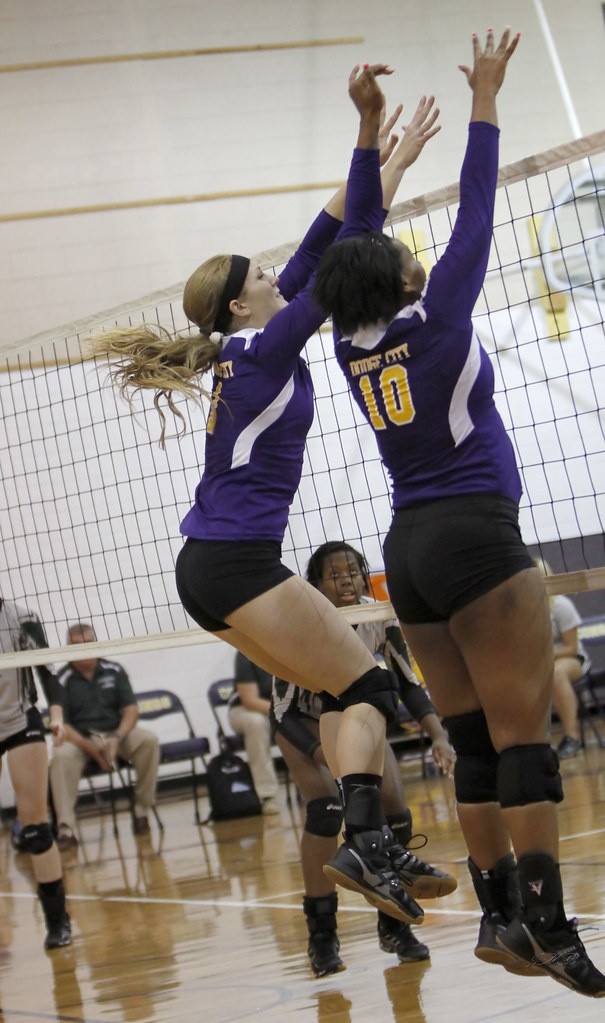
[0,598,73,951]
[49,624,160,851]
[312,29,605,998]
[529,557,593,759]
[80,95,454,923]
[274,541,456,979]
[227,651,307,814]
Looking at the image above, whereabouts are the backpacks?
[207,752,261,820]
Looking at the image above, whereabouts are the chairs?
[68,614,605,837]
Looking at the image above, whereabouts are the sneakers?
[308,931,345,978]
[556,734,585,759]
[381,832,456,898]
[44,915,71,949]
[496,914,605,1000]
[376,919,430,961]
[474,901,550,976]
[323,839,426,923]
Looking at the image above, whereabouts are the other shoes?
[133,802,153,834]
[263,797,281,815]
[56,832,77,850]
